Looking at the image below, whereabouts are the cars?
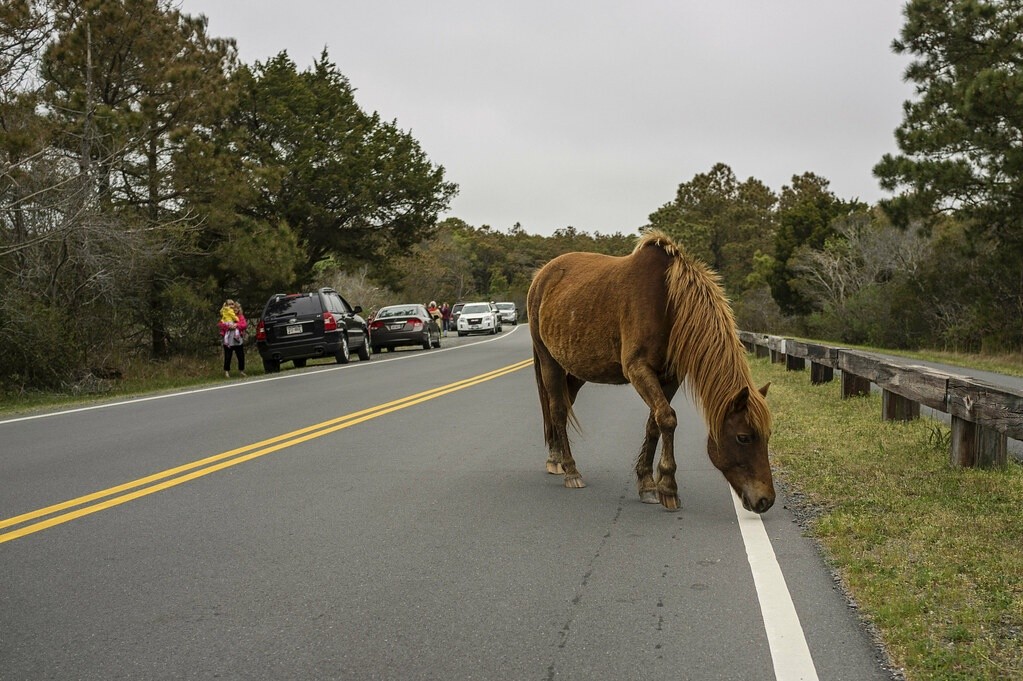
[455,302,503,337]
[495,301,519,325]
[448,303,465,331]
[370,303,442,354]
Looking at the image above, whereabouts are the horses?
[527,227,776,514]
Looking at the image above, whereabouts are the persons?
[216,299,248,379]
[427,301,451,338]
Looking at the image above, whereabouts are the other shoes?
[225,372,231,379]
[241,371,248,376]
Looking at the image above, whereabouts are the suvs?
[255,287,371,374]
